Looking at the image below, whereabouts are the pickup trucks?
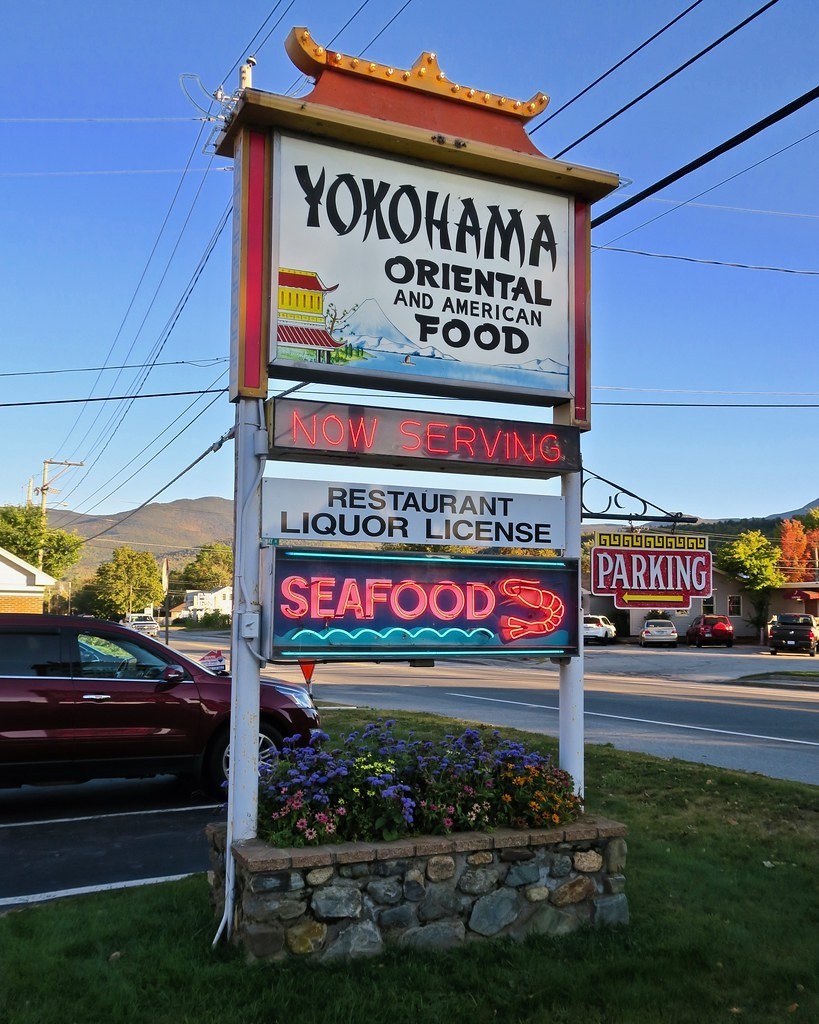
[768,613,819,657]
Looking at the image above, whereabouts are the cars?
[638,620,677,647]
[119,613,158,637]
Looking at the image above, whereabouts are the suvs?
[686,614,733,648]
[583,614,617,646]
[0,613,322,798]
